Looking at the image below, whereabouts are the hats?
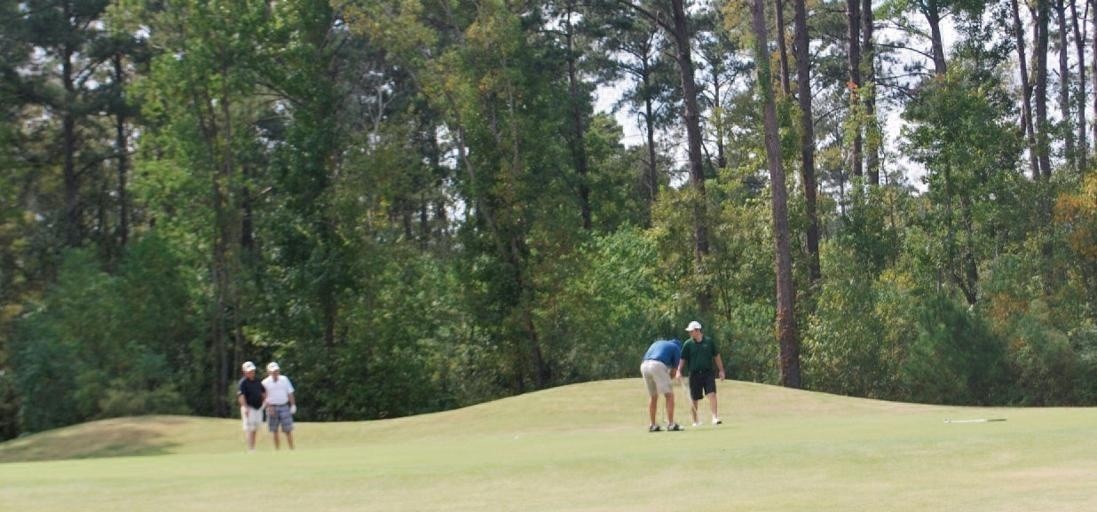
[240,361,257,373]
[684,321,701,331]
[267,362,280,372]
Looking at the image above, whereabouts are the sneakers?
[650,424,685,430]
[713,418,722,424]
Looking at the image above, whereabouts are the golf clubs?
[662,398,667,425]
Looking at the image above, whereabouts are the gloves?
[290,405,297,414]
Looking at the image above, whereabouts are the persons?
[675,321,725,426]
[640,339,685,431]
[238,361,267,452]
[261,362,297,450]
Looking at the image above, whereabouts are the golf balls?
[679,426,684,430]
